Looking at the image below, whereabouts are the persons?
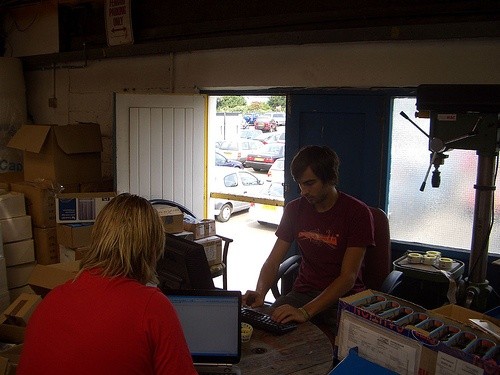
[241,144,376,342]
[15,192,199,375]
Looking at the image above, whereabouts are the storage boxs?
[0,123,112,343]
[157,206,223,268]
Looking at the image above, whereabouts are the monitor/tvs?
[160,290,241,364]
[156,231,216,290]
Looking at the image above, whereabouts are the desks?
[195,303,334,375]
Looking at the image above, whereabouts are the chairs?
[149,199,233,290]
[271,206,403,314]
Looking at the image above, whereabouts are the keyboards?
[241,305,298,337]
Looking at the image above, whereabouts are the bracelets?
[297,307,310,321]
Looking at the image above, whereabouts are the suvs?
[218,139,264,162]
[244,113,259,125]
[265,113,286,126]
[256,157,284,225]
[254,116,278,133]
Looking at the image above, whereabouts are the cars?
[240,129,285,143]
[215,167,264,222]
[245,143,285,171]
[215,150,244,169]
